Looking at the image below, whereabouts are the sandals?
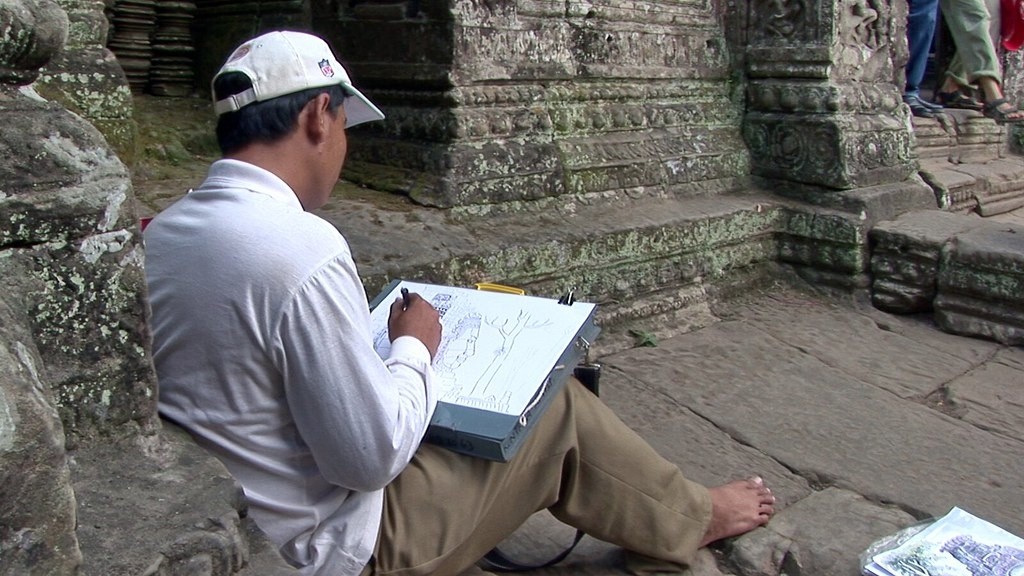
[930,89,985,111]
[981,99,1024,123]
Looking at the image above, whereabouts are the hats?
[211,30,385,130]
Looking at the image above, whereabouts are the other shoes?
[915,95,944,113]
[903,96,935,118]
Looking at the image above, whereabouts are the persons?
[942,0,1024,123]
[904,0,942,118]
[143,31,774,576]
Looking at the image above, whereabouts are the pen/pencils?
[399,286,409,308]
